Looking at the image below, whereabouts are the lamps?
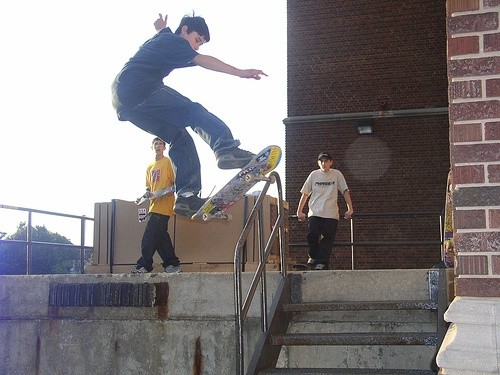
[356,119,374,136]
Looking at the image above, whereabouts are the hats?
[318,151,331,160]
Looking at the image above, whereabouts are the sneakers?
[173,191,205,217]
[216,147,255,170]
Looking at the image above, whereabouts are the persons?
[111,11,269,220]
[132,137,183,273]
[296,151,353,271]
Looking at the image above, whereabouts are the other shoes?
[307,257,316,267]
[131,264,148,273]
[314,263,328,269]
[162,263,182,272]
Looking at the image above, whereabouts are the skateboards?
[135,185,175,206]
[190,144,283,222]
[292,262,315,270]
